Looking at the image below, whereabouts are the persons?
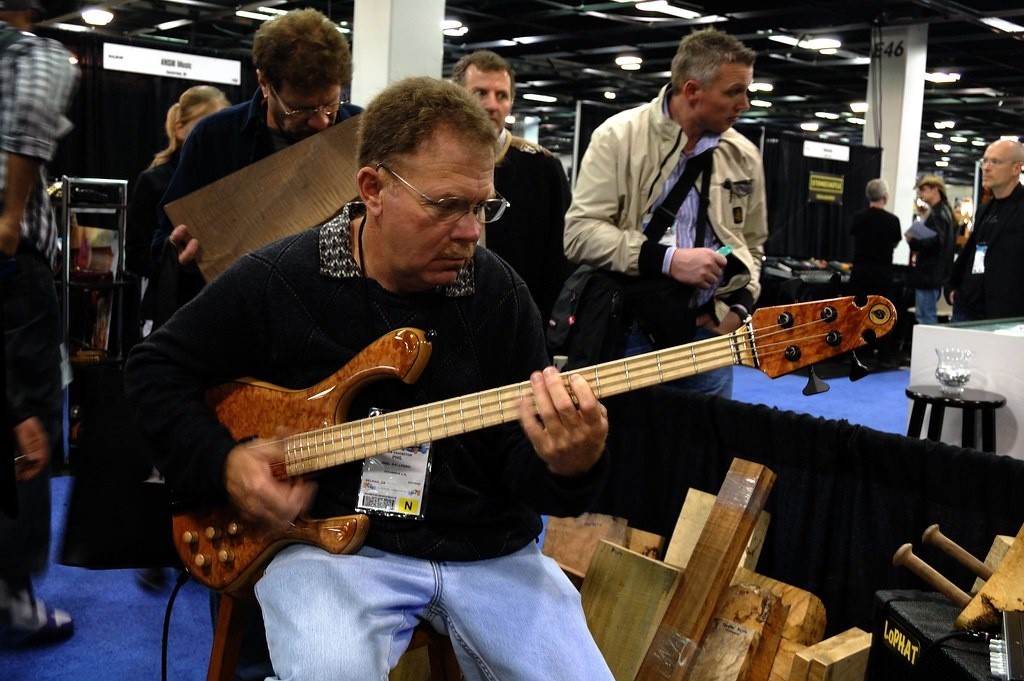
[563,26,770,400]
[0,0,82,648]
[140,87,233,173]
[153,8,367,326]
[905,172,955,326]
[118,85,614,680]
[847,175,901,370]
[957,136,1024,321]
[450,50,570,324]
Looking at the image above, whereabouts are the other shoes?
[0,580,74,648]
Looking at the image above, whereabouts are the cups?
[935,347,975,395]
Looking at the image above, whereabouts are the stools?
[907,385,1007,451]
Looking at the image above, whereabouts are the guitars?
[165,270,898,594]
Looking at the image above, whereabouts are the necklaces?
[358,214,366,276]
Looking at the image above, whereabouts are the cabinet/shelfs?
[58,174,130,466]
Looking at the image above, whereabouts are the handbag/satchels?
[547,267,621,372]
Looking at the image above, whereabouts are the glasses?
[265,79,349,121]
[979,153,1021,170]
[374,161,511,224]
